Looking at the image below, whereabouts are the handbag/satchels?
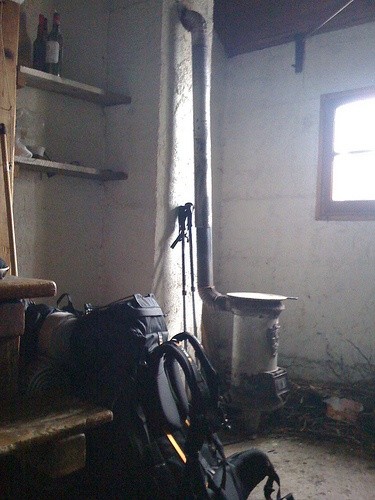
[26,291,170,415]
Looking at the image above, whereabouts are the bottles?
[45,12,64,77]
[32,14,49,72]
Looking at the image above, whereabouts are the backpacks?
[117,331,298,500]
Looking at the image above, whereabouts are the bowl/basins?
[324,396,364,426]
[0,266,10,280]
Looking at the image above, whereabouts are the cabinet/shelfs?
[14,64,131,182]
[0,1,115,499]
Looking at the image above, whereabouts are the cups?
[25,145,46,159]
